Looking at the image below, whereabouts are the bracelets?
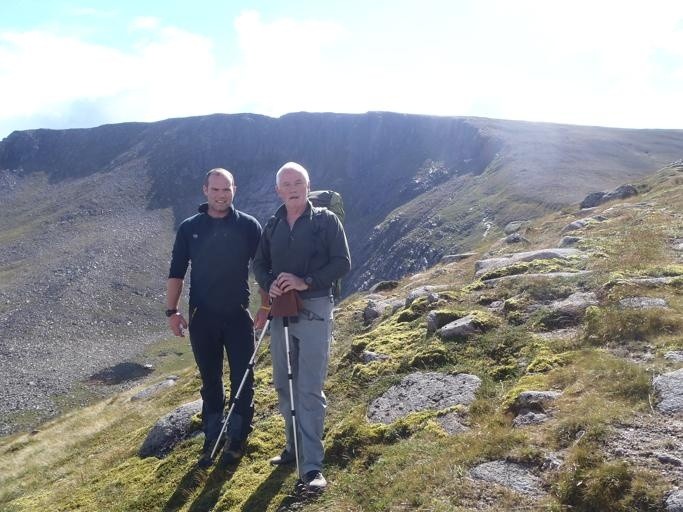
[259,305,271,310]
[165,309,178,317]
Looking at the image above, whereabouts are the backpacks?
[262,190,345,299]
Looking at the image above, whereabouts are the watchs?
[304,275,313,292]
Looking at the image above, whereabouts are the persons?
[253,162,352,487]
[164,162,270,490]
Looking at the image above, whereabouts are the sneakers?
[270,449,296,464]
[304,471,326,488]
[198,435,246,461]
